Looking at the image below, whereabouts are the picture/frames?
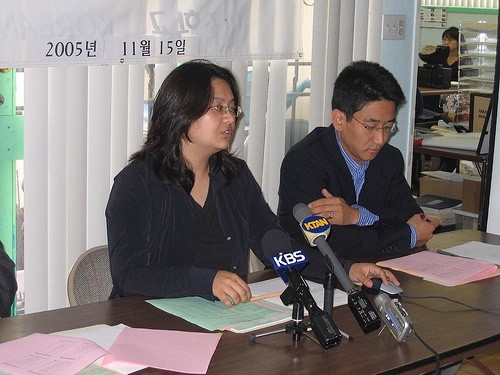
[469,92,492,133]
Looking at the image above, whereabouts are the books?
[414,130,482,226]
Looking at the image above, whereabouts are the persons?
[105,59,399,308]
[277,59,441,262]
[442,27,475,82]
[0,240,18,319]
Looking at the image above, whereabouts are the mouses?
[361,276,404,300]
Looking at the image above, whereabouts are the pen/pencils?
[419,213,431,222]
[250,291,282,302]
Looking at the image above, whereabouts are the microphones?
[261,230,340,348]
[293,202,378,332]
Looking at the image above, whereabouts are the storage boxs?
[452,203,479,231]
[462,176,481,214]
[416,194,462,226]
[420,171,468,201]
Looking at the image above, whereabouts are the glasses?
[210,102,243,118]
[340,109,398,136]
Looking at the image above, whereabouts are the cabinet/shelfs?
[456,21,497,124]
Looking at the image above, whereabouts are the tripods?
[305,255,352,340]
[248,297,323,345]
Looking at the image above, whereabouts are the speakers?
[417,67,452,89]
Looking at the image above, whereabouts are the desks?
[418,87,488,230]
[0,229,500,375]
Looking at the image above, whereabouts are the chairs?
[67,245,114,307]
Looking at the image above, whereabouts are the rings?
[328,211,332,218]
